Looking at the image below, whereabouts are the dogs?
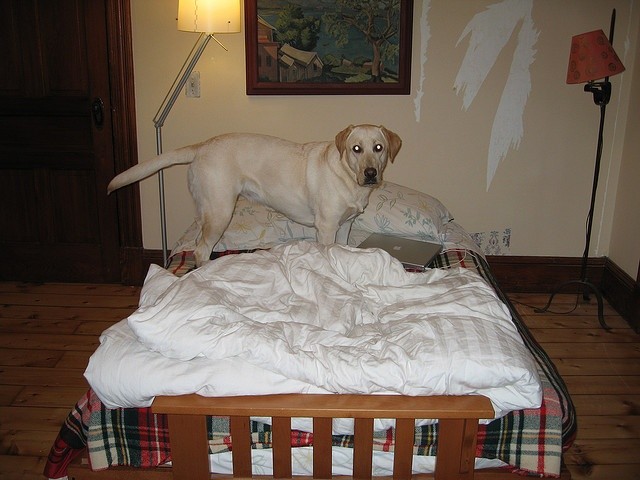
[107,123,402,268]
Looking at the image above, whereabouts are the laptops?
[356,232,443,271]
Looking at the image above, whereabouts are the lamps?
[152,1,241,266]
[534,7,626,337]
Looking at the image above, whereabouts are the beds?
[45,176,578,474]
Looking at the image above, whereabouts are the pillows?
[212,190,317,253]
[348,181,454,246]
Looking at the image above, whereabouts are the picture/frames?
[244,0,415,96]
